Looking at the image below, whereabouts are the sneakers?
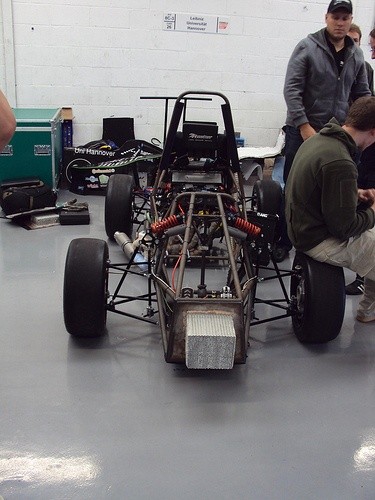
[346,276,366,295]
[356,300,374,322]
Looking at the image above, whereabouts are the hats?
[326,0,353,14]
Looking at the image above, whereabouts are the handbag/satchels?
[0,178,58,213]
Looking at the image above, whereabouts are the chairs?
[164,123,230,175]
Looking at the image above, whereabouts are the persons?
[270,0,372,262]
[344,21,374,296]
[284,96,375,323]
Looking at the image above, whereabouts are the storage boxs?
[1,109,60,194]
